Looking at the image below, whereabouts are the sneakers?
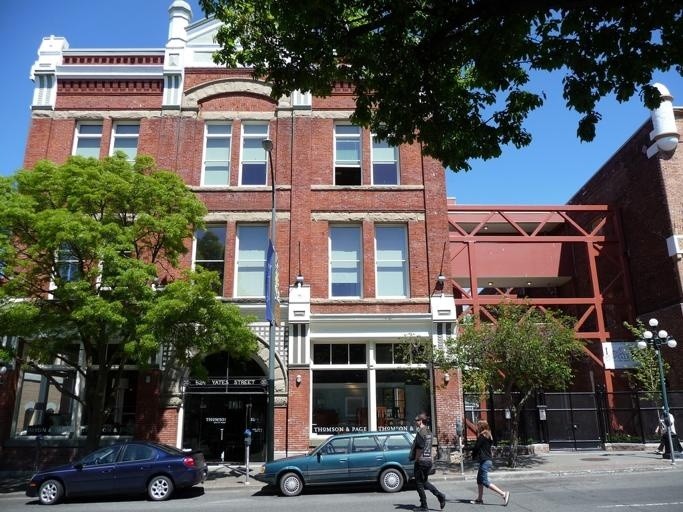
[470,491,510,506]
[414,493,445,512]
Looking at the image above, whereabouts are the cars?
[26,443,208,505]
[256,431,435,496]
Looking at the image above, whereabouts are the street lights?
[261,138,277,460]
[638,318,677,463]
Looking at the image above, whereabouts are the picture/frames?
[344,395,365,418]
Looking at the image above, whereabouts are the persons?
[408,413,446,511]
[653,412,674,454]
[659,405,683,452]
[468,419,510,507]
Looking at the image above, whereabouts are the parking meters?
[244,429,252,483]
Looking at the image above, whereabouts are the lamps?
[296,374,301,383]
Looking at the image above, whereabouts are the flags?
[260,238,282,325]
